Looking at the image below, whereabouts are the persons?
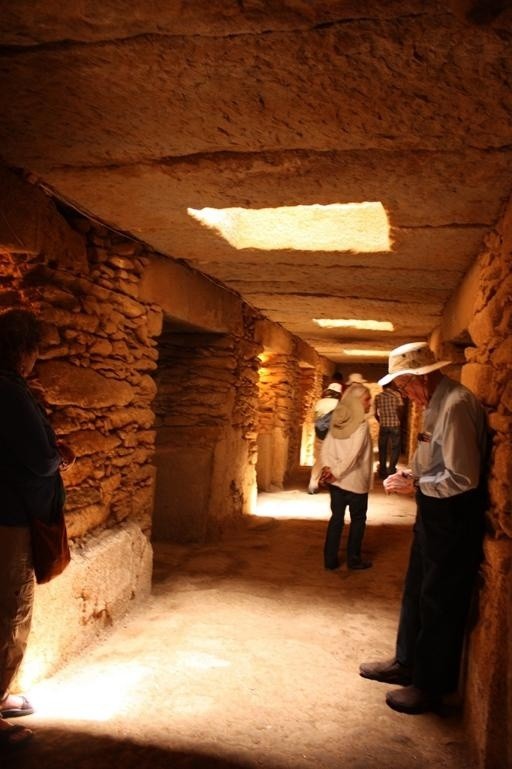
[346,374,369,388]
[360,342,491,714]
[319,382,374,569]
[333,373,343,386]
[307,383,342,495]
[373,377,405,479]
[0,310,77,750]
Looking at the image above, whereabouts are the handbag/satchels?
[315,413,333,440]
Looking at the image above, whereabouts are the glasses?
[390,375,418,393]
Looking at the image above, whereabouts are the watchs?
[413,478,421,491]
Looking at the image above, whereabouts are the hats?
[344,373,368,386]
[377,342,452,388]
[322,382,343,393]
[328,397,365,441]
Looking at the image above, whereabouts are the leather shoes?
[326,560,336,567]
[348,558,374,571]
[385,683,455,713]
[359,659,415,685]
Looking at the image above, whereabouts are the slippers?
[0,688,35,717]
[1,722,33,748]
